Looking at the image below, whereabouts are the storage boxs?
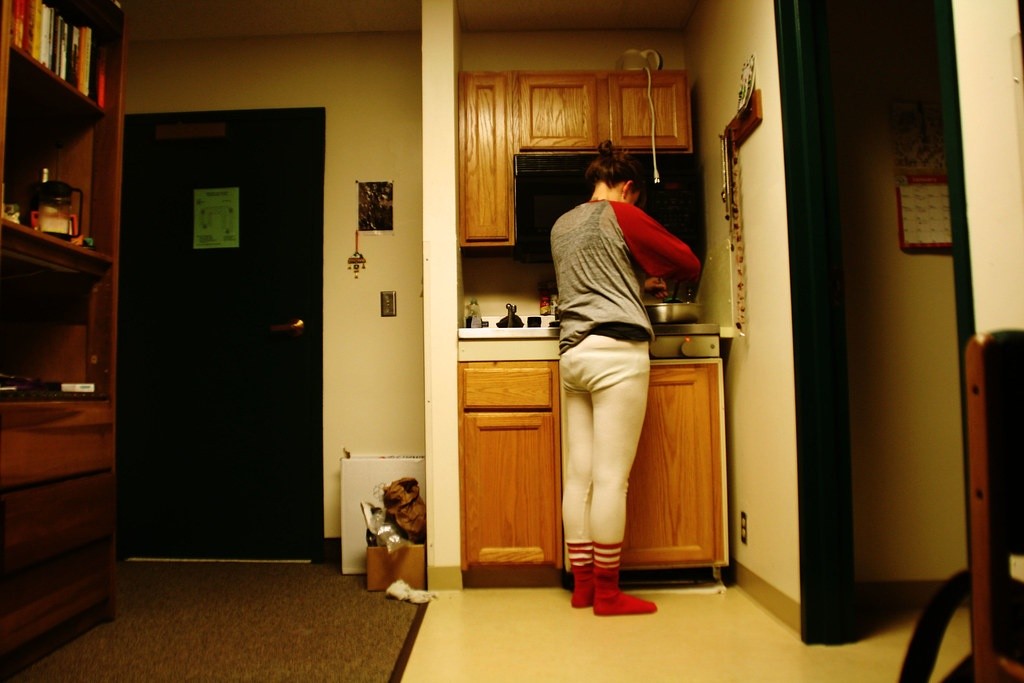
[367,545,427,592]
[339,448,427,573]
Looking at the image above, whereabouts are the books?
[10,0,107,106]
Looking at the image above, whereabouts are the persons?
[551,140,699,614]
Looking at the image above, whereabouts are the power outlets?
[741,512,747,544]
[381,291,396,316]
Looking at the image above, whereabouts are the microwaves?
[514,153,700,263]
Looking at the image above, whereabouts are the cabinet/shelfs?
[966,329,1024,683]
[516,69,694,155]
[457,69,517,256]
[0,0,130,683]
[458,358,729,588]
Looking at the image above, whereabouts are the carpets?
[0,559,429,683]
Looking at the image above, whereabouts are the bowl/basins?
[644,303,704,323]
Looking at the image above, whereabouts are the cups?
[36,180,83,242]
